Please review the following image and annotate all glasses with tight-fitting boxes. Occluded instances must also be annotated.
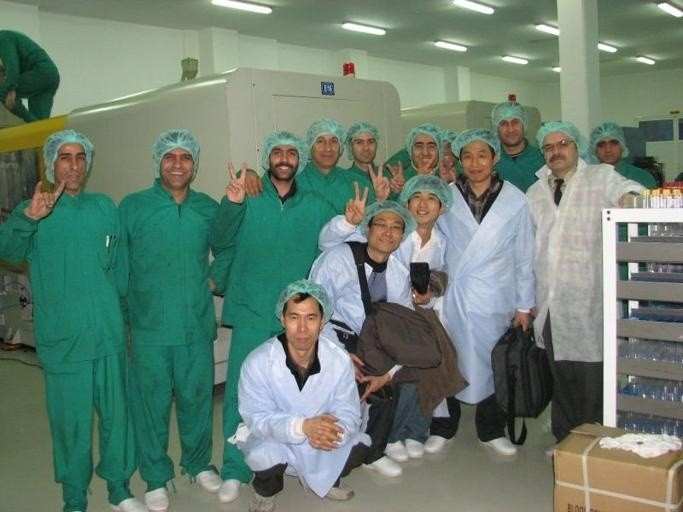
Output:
[542,139,575,153]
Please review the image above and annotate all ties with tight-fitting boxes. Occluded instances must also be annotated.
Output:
[554,179,564,206]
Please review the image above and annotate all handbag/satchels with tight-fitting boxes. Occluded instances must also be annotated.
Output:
[354,314,420,386]
[490,316,553,419]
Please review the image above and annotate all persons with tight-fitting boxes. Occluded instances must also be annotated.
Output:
[0,128,150,511]
[214,133,342,501]
[225,280,363,512]
[116,128,236,512]
[0,29,60,124]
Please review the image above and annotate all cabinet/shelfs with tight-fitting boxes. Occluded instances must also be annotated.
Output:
[600,205,682,440]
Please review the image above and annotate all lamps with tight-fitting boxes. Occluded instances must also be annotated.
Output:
[635,56,656,66]
[210,1,273,15]
[535,23,560,36]
[597,42,618,54]
[656,1,682,18]
[552,65,561,72]
[433,40,467,52]
[341,21,387,36]
[453,0,495,15]
[502,55,529,65]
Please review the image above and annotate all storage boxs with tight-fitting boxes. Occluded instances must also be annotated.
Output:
[550,422,682,512]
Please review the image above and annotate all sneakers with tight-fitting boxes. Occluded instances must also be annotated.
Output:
[217,471,255,503]
[424,435,455,456]
[405,438,425,458]
[325,478,356,502]
[195,462,223,493]
[248,490,280,512]
[104,492,150,512]
[384,440,408,462]
[361,453,403,478]
[143,487,169,512]
[476,434,518,457]
